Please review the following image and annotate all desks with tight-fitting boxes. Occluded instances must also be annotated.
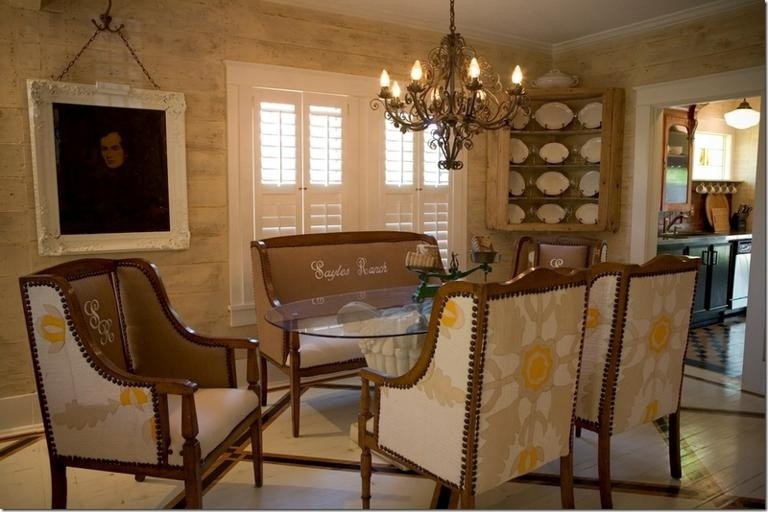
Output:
[264,282,476,472]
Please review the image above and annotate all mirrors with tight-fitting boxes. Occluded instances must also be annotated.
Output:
[660,109,691,213]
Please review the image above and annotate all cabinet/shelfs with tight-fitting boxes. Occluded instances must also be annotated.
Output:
[688,243,733,327]
[485,85,627,234]
[658,248,688,255]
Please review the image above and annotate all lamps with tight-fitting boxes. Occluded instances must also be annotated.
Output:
[724,98,760,129]
[368,0,531,172]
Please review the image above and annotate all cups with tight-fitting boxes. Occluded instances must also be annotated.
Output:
[695,182,737,194]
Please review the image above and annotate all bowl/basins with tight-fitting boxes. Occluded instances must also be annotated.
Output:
[669,146,684,155]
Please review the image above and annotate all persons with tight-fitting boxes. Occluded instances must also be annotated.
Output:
[81,129,161,232]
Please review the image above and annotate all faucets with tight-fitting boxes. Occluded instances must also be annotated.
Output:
[661,211,688,233]
[662,236,688,241]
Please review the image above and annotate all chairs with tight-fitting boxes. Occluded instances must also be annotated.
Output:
[507,236,606,278]
[356,266,587,510]
[20,260,263,510]
[576,253,701,509]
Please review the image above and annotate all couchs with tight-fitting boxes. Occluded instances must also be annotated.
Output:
[250,230,447,438]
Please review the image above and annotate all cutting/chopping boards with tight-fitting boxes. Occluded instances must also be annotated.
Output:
[711,207,732,233]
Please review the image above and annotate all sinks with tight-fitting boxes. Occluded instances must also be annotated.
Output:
[658,231,712,239]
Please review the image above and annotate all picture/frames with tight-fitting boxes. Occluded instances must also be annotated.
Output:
[26,80,191,256]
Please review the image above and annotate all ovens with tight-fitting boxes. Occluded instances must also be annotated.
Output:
[727,238,752,310]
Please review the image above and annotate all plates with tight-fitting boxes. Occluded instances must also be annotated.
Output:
[506,101,603,223]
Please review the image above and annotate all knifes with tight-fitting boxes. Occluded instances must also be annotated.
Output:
[735,202,753,218]
